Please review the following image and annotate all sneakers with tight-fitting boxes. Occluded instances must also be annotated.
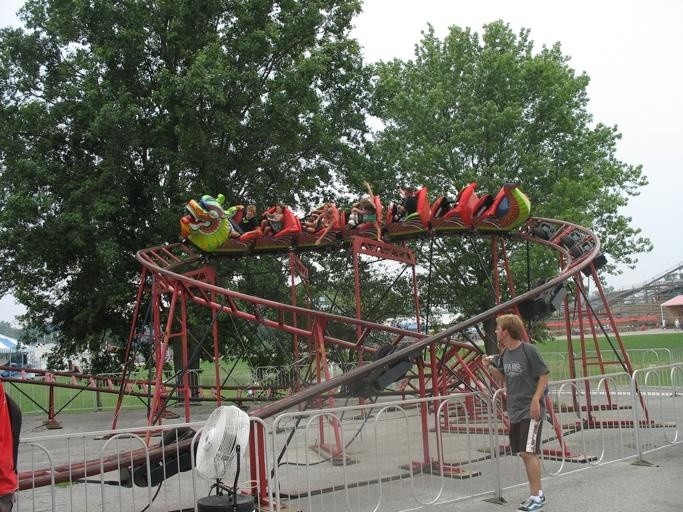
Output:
[516,496,546,512]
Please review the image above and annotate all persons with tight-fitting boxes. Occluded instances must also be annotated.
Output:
[349,184,418,228]
[262,203,284,236]
[234,204,260,239]
[302,203,334,233]
[480,314,550,511]
